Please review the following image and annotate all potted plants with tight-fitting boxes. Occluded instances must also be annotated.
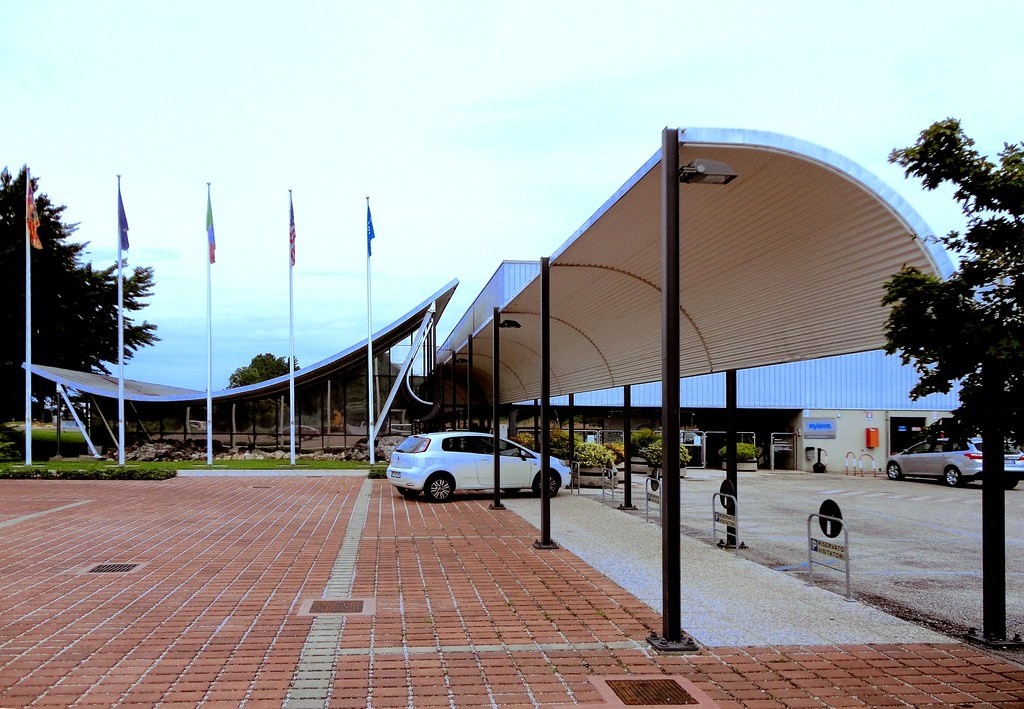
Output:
[570,428,692,487]
[717,441,763,471]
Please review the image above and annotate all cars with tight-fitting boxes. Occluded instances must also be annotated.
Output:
[386,431,572,504]
[271,425,320,441]
[886,436,1024,490]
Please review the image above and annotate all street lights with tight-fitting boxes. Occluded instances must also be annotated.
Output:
[646,127,735,653]
[450,352,466,432]
[486,307,521,509]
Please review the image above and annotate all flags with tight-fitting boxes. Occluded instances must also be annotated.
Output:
[206,195,216,264]
[367,203,375,258]
[119,191,129,251]
[289,191,296,267]
[25,173,42,249]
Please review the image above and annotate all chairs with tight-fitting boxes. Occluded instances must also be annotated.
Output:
[942,444,954,451]
